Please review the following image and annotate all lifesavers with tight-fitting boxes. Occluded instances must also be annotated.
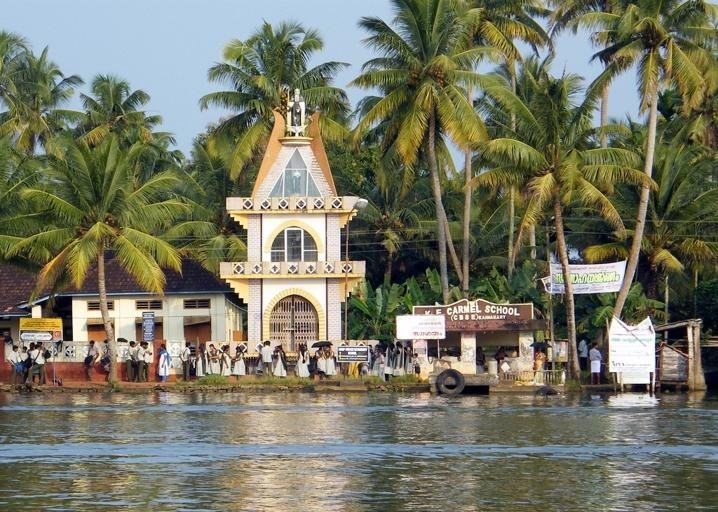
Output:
[436,369,464,395]
